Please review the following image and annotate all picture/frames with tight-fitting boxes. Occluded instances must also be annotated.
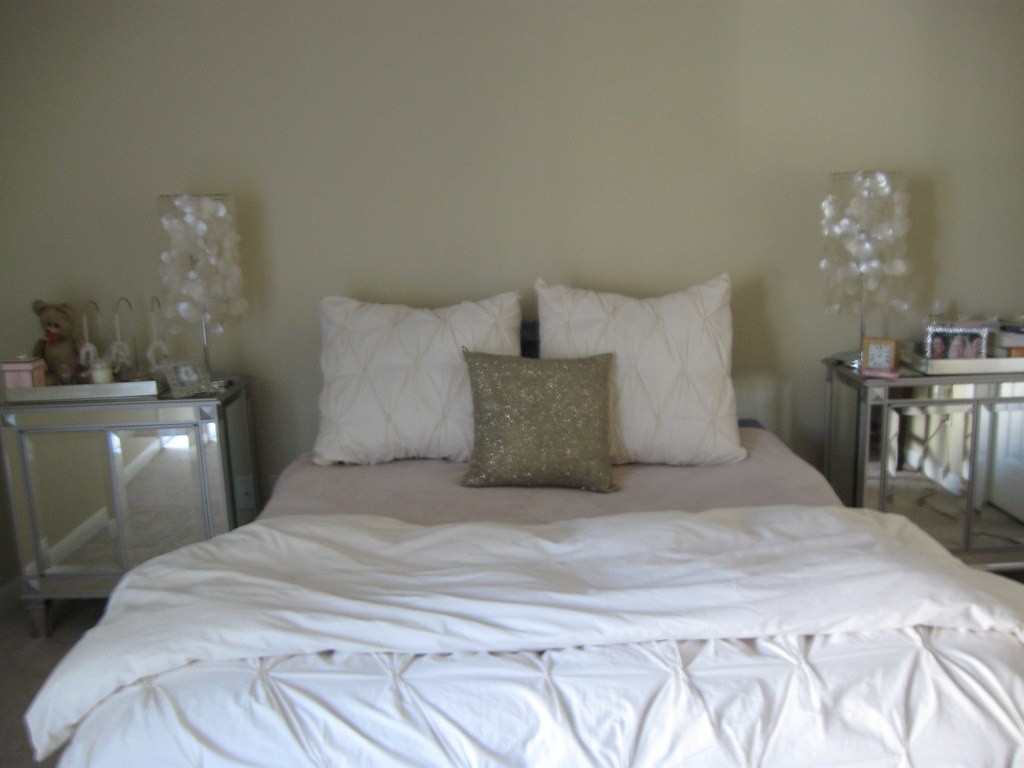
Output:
[924,326,988,360]
[160,353,213,398]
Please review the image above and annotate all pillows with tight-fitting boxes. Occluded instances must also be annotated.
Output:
[532,272,751,468]
[459,344,622,494]
[309,289,523,468]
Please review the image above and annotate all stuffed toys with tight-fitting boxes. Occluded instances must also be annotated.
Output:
[32,300,82,386]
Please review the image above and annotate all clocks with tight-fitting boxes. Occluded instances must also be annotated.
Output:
[860,337,898,372]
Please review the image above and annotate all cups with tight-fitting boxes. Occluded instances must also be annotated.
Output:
[88,358,114,384]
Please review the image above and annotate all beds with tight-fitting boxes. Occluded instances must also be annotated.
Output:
[21,374,1024,768]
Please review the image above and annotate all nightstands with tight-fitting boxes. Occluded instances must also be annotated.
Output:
[0,376,262,639]
[818,348,1023,573]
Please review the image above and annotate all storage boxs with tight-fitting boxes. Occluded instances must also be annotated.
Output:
[0,354,47,387]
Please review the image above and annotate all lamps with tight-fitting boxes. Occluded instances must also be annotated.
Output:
[157,190,250,388]
[815,171,912,367]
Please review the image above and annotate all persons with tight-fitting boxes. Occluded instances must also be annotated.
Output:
[931,333,982,358]
